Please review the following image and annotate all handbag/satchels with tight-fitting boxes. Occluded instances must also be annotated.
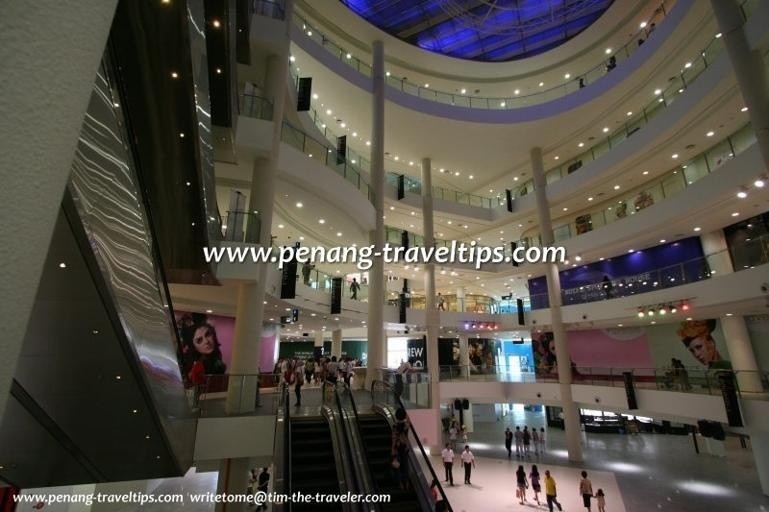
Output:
[516,487,521,499]
[296,368,304,385]
[535,484,541,492]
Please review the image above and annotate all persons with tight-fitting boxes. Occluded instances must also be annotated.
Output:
[189,323,226,384]
[246,468,257,495]
[438,293,445,311]
[391,408,412,490]
[442,421,605,512]
[256,355,362,408]
[256,467,269,512]
[302,258,315,284]
[533,333,557,373]
[395,357,417,405]
[350,277,361,300]
[676,319,723,369]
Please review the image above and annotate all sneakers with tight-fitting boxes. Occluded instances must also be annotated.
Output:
[294,401,301,406]
